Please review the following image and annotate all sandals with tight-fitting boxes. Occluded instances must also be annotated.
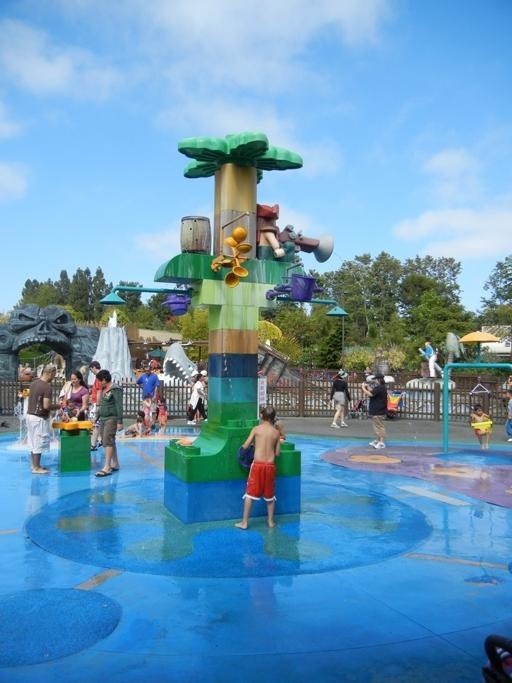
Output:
[91,445,97,451]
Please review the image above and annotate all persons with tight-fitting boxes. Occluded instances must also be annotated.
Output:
[329,370,352,430]
[16,358,209,453]
[504,389,512,443]
[480,630,512,683]
[419,341,433,361]
[361,373,388,450]
[233,402,278,529]
[24,360,63,475]
[469,404,492,452]
[92,370,125,477]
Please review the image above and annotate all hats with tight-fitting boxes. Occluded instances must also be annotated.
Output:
[371,374,384,380]
[338,371,348,378]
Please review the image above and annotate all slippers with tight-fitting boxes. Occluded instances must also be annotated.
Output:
[95,470,112,476]
[110,466,119,471]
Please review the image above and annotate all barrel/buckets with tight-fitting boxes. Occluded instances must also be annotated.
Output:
[179,215,212,255]
[291,273,317,302]
[238,444,255,469]
[167,293,188,316]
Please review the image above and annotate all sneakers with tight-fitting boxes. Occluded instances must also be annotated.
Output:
[374,442,385,448]
[341,422,348,427]
[187,420,196,424]
[330,423,340,429]
[369,440,378,446]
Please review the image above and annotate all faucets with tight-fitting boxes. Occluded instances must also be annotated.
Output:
[221,211,250,229]
